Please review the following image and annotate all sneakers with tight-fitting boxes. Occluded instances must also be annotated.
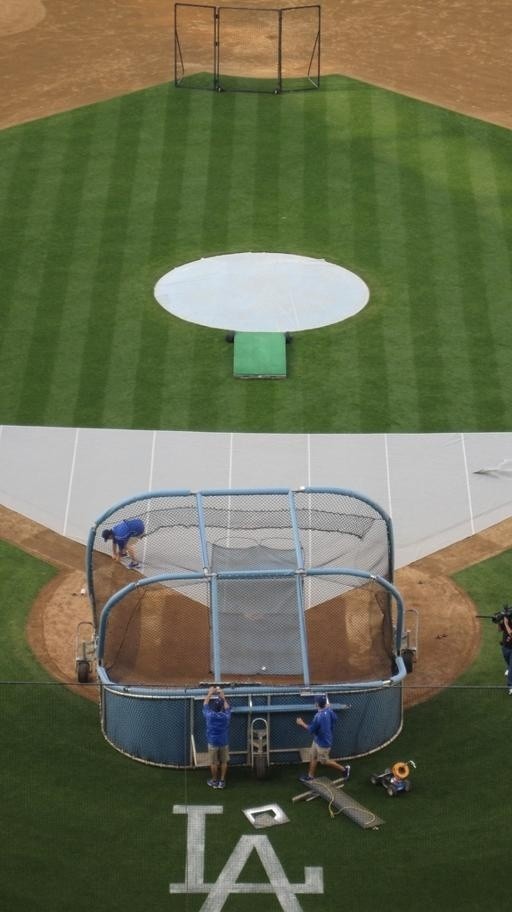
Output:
[122,552,129,558]
[298,775,315,782]
[218,780,225,789]
[127,561,142,569]
[342,765,351,779]
[206,778,218,788]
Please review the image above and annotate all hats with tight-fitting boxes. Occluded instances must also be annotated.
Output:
[213,697,225,708]
[314,693,327,705]
[101,528,114,541]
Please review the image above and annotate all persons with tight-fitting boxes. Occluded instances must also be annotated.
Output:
[296,693,350,783]
[202,686,231,790]
[101,517,145,570]
[497,606,512,697]
